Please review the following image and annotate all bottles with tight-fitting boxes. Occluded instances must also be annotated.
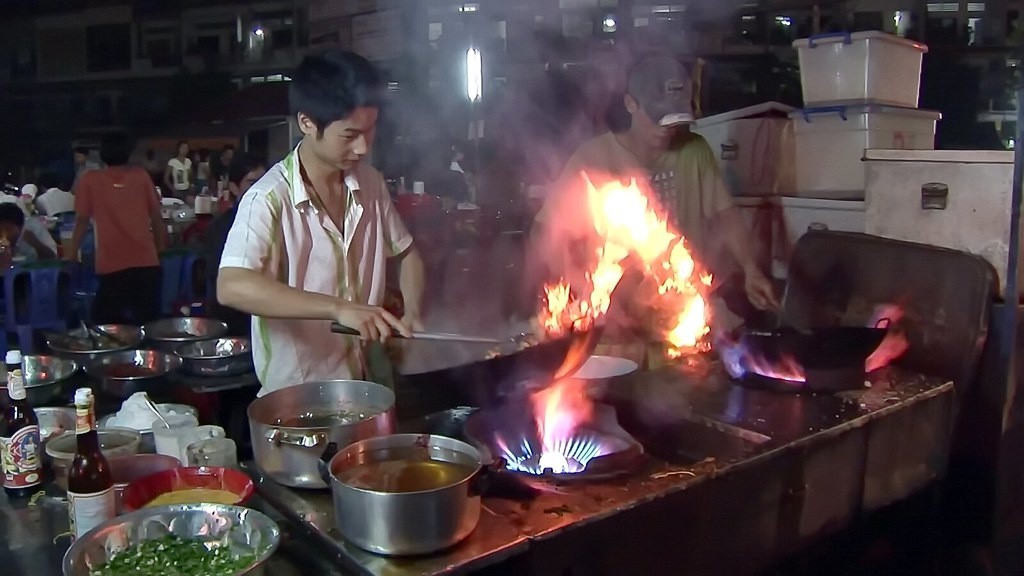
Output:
[67,388,116,544]
[0,228,12,274]
[0,351,46,499]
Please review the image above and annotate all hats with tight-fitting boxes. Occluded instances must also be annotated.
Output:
[628,53,696,128]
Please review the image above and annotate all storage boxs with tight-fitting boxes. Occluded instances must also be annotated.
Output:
[691,31,1018,305]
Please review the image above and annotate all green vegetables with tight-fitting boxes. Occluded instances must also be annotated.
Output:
[88,532,255,576]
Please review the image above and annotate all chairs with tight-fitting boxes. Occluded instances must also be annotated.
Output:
[0,207,201,352]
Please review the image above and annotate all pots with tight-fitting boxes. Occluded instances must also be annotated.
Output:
[247,380,397,489]
[719,318,891,384]
[318,432,502,555]
[398,318,606,408]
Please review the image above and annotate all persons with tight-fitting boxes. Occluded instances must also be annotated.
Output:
[216,45,432,397]
[0,104,523,371]
[527,55,780,330]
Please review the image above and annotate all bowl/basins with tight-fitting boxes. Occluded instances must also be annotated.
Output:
[1,356,78,405]
[47,424,142,491]
[120,466,254,512]
[62,502,279,576]
[96,405,198,454]
[173,338,254,376]
[82,348,182,401]
[145,317,229,354]
[45,324,144,365]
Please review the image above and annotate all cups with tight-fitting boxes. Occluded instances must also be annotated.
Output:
[178,425,225,466]
[186,439,237,466]
[222,189,231,202]
[153,415,199,462]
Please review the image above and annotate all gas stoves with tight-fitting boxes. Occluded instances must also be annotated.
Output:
[395,295,953,539]
